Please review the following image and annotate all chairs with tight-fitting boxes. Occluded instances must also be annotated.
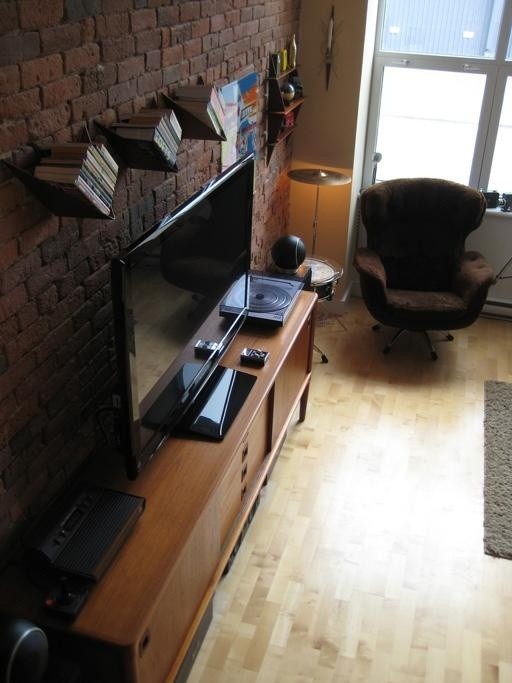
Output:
[352,177,494,362]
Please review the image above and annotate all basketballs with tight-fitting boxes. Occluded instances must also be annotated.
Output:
[272,235,305,269]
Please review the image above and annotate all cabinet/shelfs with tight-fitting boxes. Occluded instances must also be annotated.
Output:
[267,50,306,166]
[32,276,320,683]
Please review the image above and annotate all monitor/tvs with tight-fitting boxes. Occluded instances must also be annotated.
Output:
[109,151,258,482]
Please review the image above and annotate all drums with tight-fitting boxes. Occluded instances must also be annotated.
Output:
[271,254,343,303]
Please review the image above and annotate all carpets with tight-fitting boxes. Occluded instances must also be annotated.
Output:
[483,380,512,561]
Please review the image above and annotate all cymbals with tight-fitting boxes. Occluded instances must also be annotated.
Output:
[287,169,352,186]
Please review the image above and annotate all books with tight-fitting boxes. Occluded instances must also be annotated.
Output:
[29,82,227,216]
